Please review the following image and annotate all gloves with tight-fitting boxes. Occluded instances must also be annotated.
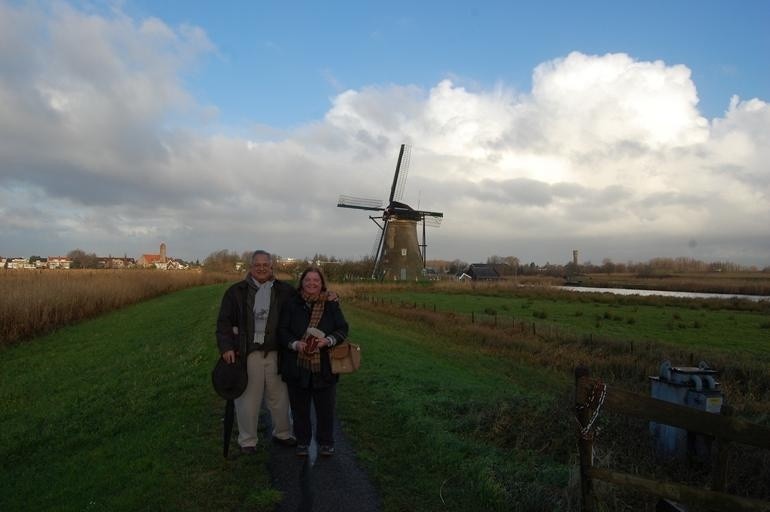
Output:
[303,327,326,355]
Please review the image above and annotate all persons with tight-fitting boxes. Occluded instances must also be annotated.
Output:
[275,266,350,457]
[215,250,341,456]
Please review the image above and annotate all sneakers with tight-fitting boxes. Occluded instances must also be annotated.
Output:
[238,434,337,457]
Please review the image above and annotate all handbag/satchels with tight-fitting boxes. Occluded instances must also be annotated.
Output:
[211,352,248,401]
[328,337,361,374]
